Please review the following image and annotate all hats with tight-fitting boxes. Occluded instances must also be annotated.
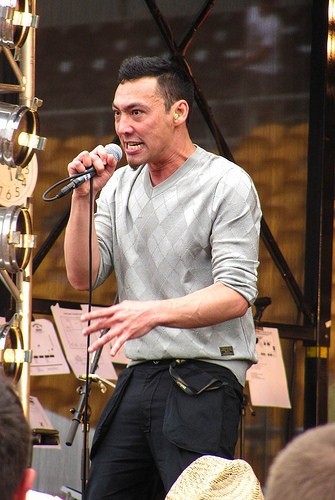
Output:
[165,455,264,500]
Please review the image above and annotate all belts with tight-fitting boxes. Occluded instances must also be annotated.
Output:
[148,360,224,369]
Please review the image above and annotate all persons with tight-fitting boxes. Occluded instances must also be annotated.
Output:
[64,52,263,500]
[228,0,288,121]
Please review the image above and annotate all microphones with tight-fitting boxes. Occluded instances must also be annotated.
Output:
[56,143,123,199]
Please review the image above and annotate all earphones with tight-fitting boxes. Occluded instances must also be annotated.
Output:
[174,112,179,120]
